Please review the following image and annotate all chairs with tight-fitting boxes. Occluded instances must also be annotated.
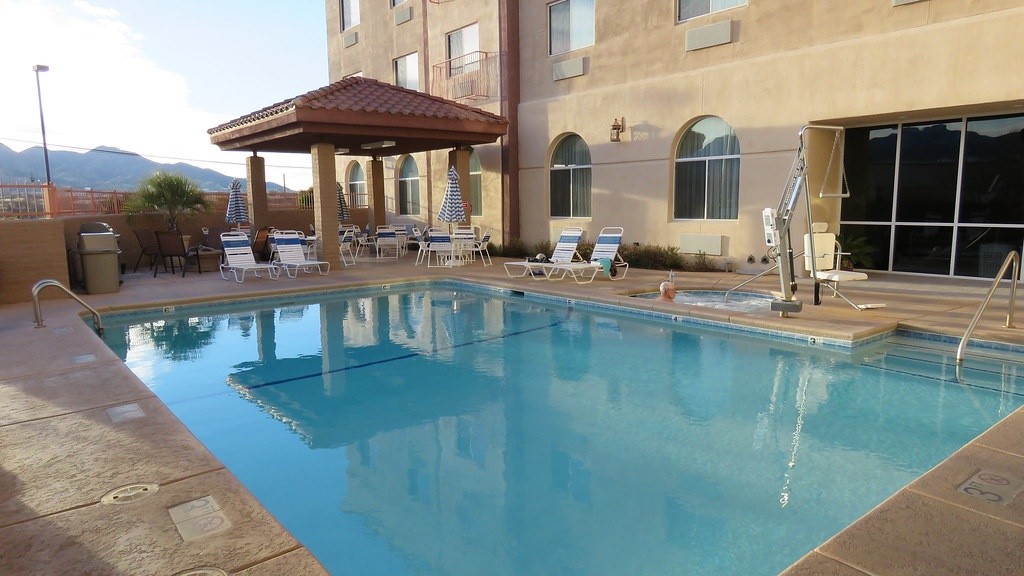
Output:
[804,222,887,312]
[154,231,202,277]
[504,227,588,281]
[542,226,629,285]
[220,224,493,283]
[132,229,183,273]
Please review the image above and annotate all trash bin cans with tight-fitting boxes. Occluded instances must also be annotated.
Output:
[75,231,122,295]
[77,221,124,285]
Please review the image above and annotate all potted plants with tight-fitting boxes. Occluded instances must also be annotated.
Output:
[123,172,215,268]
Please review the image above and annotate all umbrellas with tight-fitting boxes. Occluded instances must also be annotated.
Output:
[225,177,249,232]
[336,182,350,227]
[437,166,466,235]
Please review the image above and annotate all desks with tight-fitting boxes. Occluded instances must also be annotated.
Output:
[183,251,222,272]
[432,235,476,266]
[301,236,316,259]
[375,231,407,257]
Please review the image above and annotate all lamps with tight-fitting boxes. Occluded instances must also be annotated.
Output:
[610,116,624,142]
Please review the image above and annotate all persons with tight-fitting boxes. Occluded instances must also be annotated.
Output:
[656,282,676,303]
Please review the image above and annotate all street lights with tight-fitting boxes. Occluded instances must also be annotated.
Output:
[32,64,51,185]
